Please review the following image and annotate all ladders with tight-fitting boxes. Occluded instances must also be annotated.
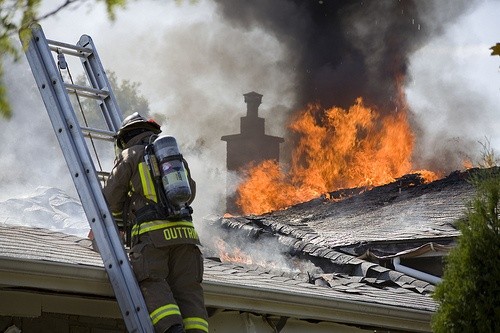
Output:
[18,22,154,333]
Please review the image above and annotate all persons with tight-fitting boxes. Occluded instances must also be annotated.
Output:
[102,111,212,332]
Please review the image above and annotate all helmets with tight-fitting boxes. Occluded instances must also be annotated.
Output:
[112,111,162,150]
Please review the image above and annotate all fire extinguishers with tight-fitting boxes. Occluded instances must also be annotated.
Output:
[145,134,193,217]
